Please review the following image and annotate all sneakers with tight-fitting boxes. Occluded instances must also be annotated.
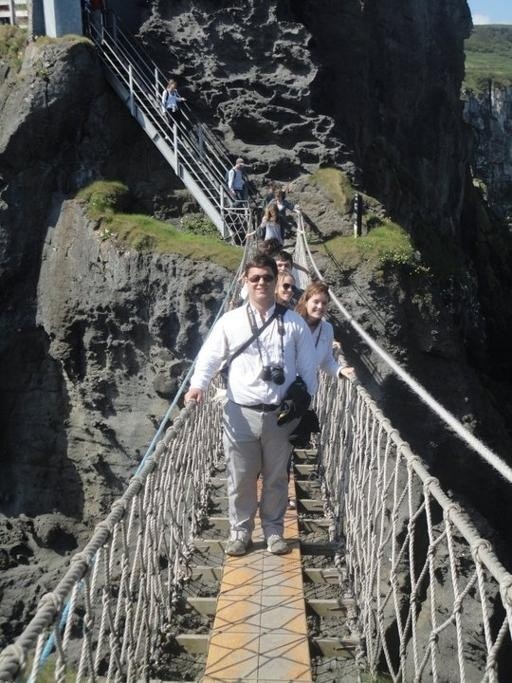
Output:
[265,532,289,554]
[224,530,252,556]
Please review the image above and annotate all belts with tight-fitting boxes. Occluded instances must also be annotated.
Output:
[231,404,279,413]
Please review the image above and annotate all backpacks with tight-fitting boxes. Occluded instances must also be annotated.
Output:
[224,169,236,185]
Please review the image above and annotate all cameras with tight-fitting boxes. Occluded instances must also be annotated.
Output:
[262,365,285,386]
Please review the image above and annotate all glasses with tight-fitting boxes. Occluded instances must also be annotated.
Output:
[280,283,297,292]
[247,275,276,283]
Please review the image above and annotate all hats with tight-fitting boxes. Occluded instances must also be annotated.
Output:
[237,158,245,166]
[276,385,311,427]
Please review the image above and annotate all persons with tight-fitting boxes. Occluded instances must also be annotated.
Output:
[245,206,305,254]
[161,79,187,140]
[259,185,283,216]
[272,252,293,276]
[273,273,297,313]
[266,191,298,241]
[183,255,321,555]
[89,0,106,46]
[228,158,246,212]
[266,284,355,510]
[258,239,312,277]
